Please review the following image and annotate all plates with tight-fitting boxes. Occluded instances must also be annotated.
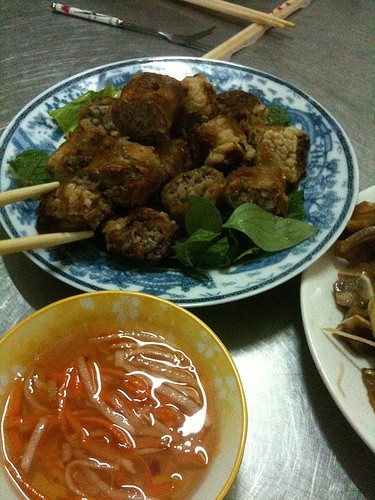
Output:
[299,183,375,455]
[1,56,361,308]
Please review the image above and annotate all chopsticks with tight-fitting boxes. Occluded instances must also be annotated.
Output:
[199,0,308,61]
[183,0,295,29]
[1,181,95,258]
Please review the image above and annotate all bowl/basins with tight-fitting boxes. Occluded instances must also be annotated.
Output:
[0,290,247,500]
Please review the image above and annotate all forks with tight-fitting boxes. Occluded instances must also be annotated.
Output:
[51,3,218,47]
